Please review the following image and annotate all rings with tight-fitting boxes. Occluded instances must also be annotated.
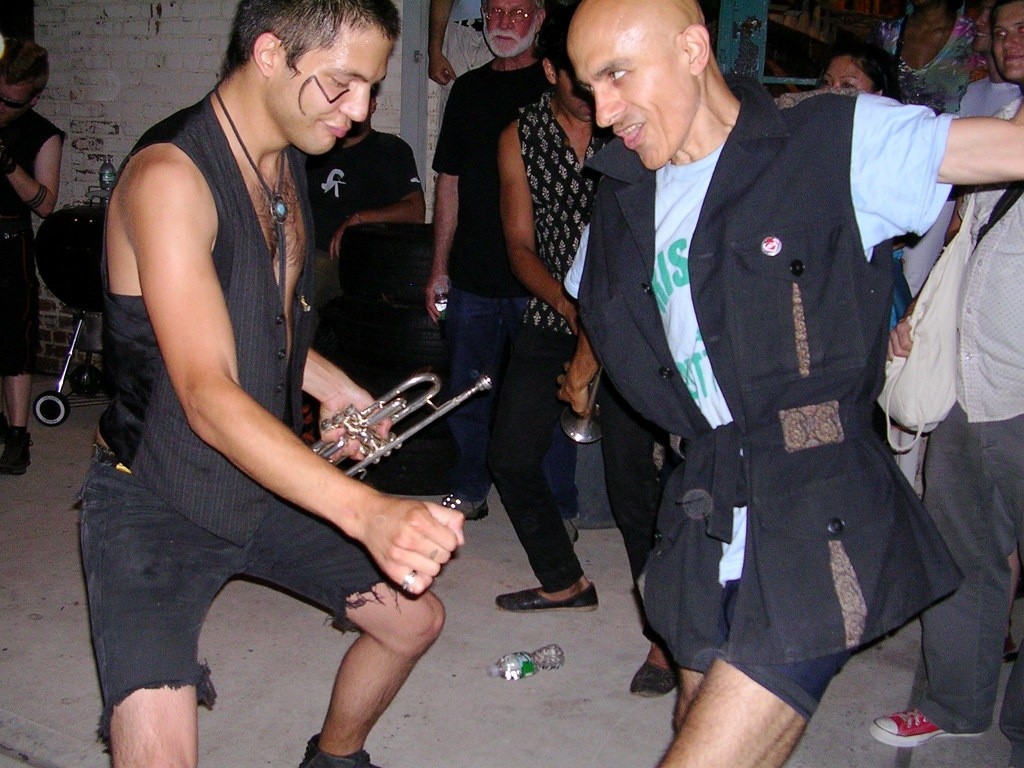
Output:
[430,547,439,560]
[401,570,417,591]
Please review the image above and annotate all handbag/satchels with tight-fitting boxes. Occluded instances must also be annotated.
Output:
[877,190,976,453]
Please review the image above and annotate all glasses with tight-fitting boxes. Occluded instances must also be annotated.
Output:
[0,88,39,108]
[484,7,538,22]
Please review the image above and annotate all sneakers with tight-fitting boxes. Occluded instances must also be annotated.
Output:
[0,411,9,444]
[869,707,984,747]
[0,429,33,475]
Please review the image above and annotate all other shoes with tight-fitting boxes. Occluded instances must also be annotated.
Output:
[495,581,599,613]
[562,517,579,548]
[446,499,489,521]
[630,660,677,697]
[298,732,381,768]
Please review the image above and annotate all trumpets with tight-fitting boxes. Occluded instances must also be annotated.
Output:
[308,369,494,478]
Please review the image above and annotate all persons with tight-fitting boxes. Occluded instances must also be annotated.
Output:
[0,38,68,474]
[76,1,464,768]
[569,1,1024,768]
[302,1,685,696]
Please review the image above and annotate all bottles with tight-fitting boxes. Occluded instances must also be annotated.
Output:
[433,284,448,342]
[486,642,565,681]
[99,155,117,206]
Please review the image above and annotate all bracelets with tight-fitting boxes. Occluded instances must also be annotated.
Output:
[354,213,362,226]
[0,156,17,174]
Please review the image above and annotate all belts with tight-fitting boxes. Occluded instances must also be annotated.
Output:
[457,19,482,32]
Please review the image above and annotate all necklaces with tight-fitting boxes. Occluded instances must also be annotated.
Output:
[215,83,287,224]
